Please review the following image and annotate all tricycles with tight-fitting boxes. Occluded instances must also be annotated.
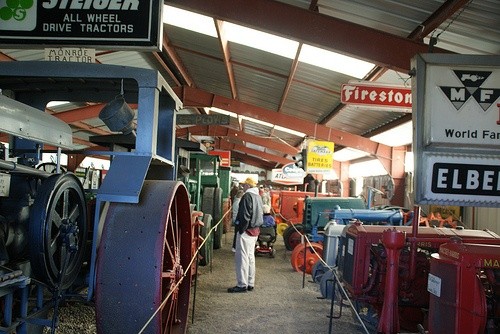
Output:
[256,222,276,258]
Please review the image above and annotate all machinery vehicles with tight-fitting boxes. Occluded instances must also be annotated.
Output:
[259,179,500,334]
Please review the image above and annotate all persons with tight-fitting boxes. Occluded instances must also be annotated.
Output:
[228,177,263,292]
[232,190,243,252]
[230,180,272,226]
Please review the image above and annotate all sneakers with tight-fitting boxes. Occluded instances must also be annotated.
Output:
[231,248,236,253]
[227,286,254,293]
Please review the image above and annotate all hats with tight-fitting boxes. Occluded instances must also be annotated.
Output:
[263,205,270,213]
[239,178,256,186]
